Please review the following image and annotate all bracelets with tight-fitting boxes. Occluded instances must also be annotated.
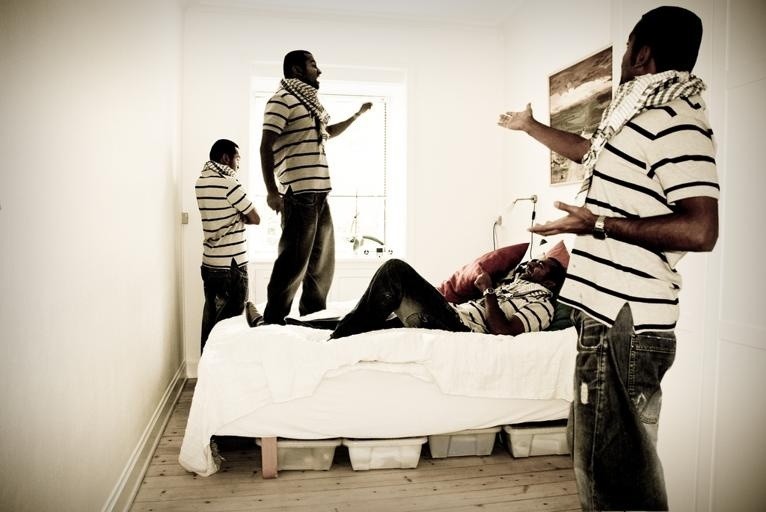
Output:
[355,113,361,116]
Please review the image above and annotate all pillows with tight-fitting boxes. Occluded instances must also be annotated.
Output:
[438,240,572,304]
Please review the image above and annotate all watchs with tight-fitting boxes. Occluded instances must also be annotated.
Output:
[592,215,607,240]
[483,287,496,296]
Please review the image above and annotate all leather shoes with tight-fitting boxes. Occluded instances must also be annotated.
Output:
[246,302,263,327]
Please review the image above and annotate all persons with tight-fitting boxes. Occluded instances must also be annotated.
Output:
[499,4,723,512]
[244,257,567,341]
[193,136,261,359]
[262,48,376,327]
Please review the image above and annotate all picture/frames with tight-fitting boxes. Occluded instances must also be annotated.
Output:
[546,45,613,187]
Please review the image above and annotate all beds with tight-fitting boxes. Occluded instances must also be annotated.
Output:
[193,303,577,479]
